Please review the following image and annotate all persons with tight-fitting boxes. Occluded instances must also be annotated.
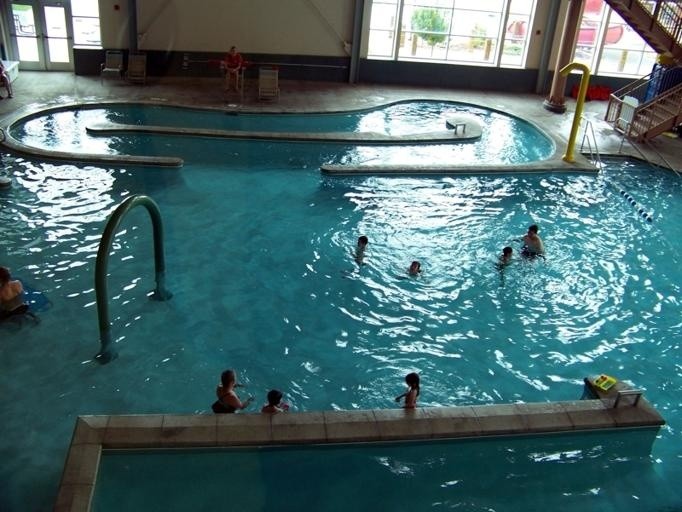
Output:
[395,261,423,280]
[261,389,291,413]
[1,266,42,330]
[494,246,521,287]
[211,368,256,413]
[223,46,242,94]
[339,235,368,280]
[393,371,421,408]
[0,58,14,99]
[509,223,549,266]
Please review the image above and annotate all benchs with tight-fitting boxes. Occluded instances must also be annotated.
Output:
[572,82,612,100]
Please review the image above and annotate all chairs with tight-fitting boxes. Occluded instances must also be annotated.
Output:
[258,68,280,103]
[125,56,146,85]
[220,60,248,96]
[100,51,124,86]
